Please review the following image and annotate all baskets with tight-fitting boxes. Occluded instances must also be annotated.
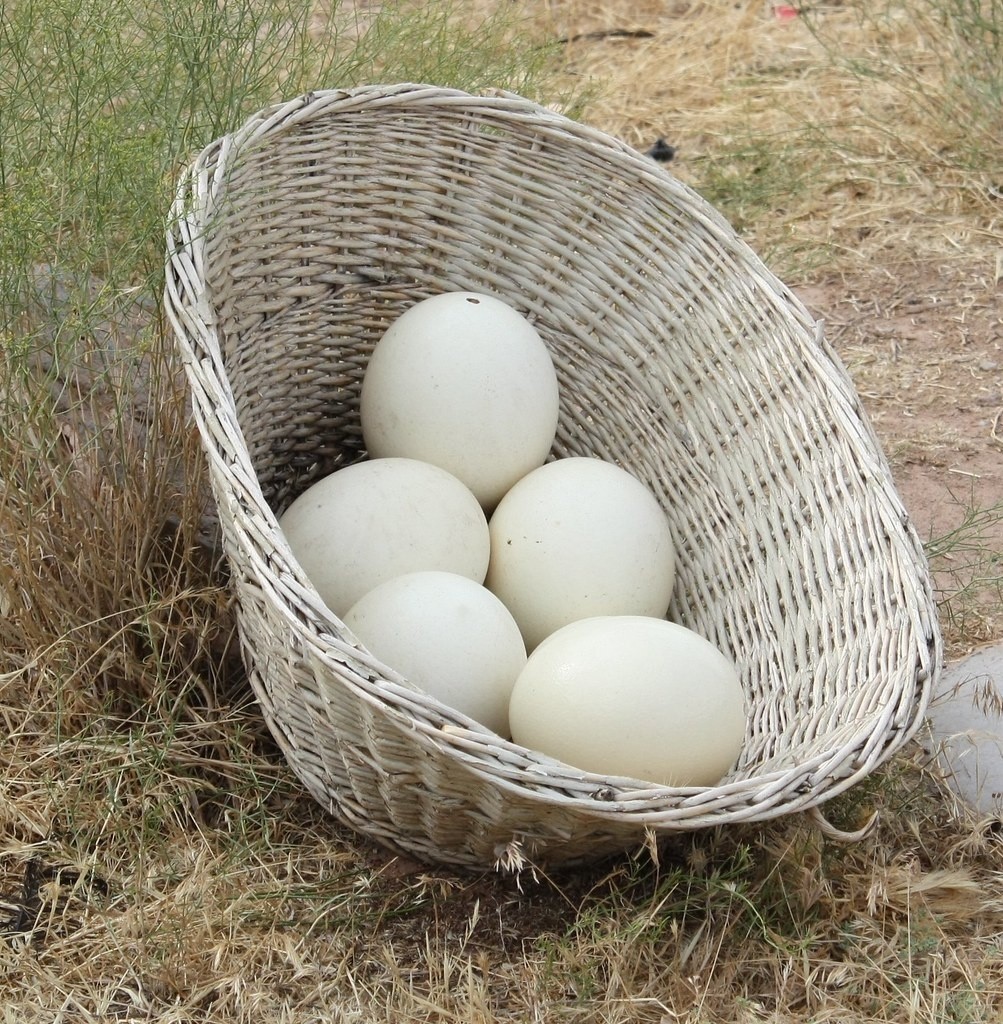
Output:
[163,83,943,875]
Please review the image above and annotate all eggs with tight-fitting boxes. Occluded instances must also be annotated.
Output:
[279,291,746,786]
